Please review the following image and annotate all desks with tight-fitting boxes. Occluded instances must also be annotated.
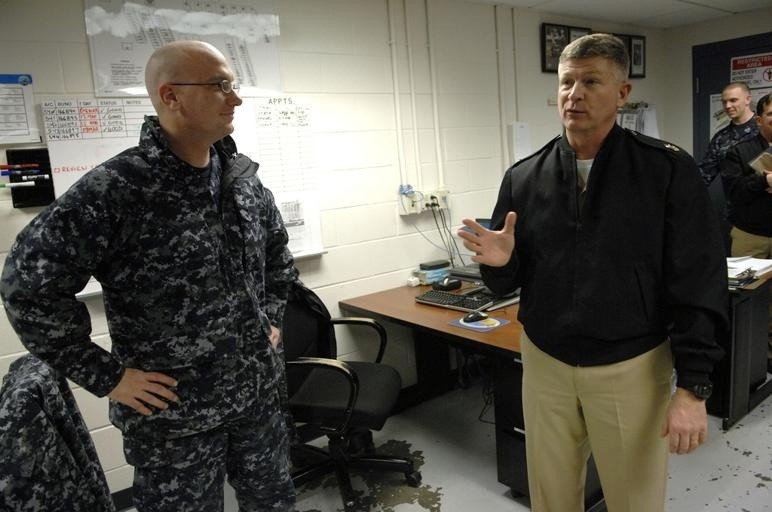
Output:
[338,280,607,512]
[706,255,772,431]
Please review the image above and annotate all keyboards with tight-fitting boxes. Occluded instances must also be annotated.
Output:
[414,289,494,313]
[469,292,520,311]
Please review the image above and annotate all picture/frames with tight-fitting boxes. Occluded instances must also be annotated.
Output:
[540,22,646,79]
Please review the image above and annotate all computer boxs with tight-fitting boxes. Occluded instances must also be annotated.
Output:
[493,375,602,504]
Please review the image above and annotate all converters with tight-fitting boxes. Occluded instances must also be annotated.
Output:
[407,276,421,287]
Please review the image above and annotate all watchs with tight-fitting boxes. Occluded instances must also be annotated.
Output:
[677,381,711,400]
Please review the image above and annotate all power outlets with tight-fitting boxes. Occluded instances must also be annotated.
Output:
[422,192,440,212]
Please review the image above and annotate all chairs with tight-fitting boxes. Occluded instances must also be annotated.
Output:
[284,283,422,512]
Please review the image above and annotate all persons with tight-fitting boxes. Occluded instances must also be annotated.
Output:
[457,31,728,511]
[698,83,761,206]
[723,95,772,259]
[0,38,299,511]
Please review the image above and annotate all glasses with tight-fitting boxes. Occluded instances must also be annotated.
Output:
[166,76,235,95]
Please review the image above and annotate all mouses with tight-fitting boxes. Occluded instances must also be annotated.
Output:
[463,310,488,322]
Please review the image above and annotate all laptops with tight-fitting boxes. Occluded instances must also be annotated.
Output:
[450,218,492,279]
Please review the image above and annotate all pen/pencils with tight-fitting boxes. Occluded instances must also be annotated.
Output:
[0,164,50,188]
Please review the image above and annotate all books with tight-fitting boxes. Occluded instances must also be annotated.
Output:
[725,256,772,291]
